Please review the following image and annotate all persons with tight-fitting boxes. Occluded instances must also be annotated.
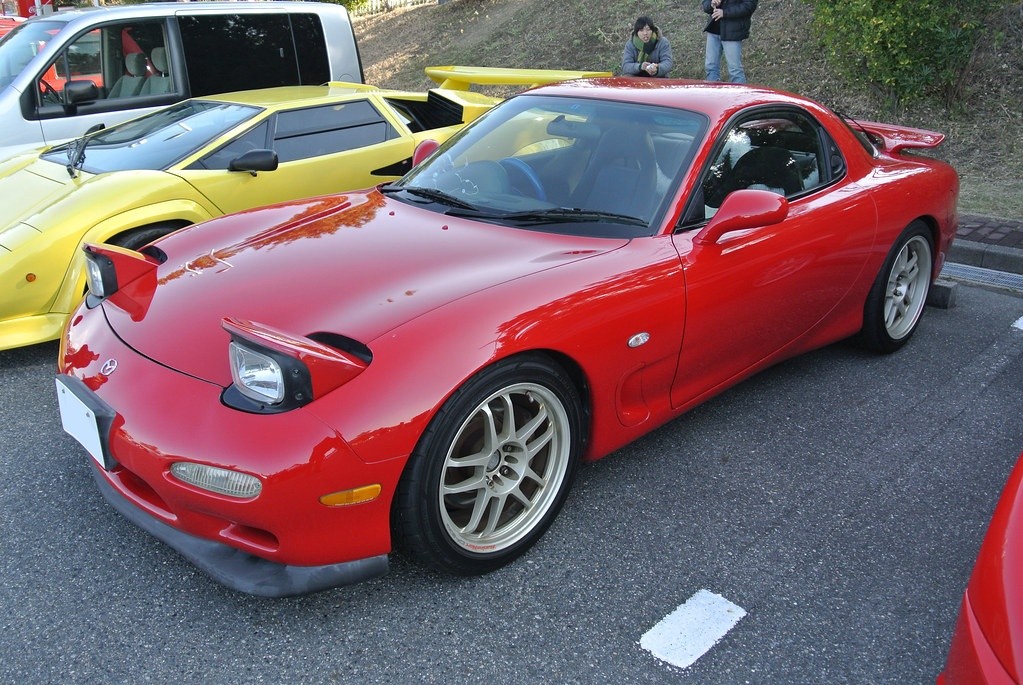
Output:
[622,16,672,79]
[702,0,760,85]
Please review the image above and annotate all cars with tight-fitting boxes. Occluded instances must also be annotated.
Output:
[54,76,958,598]
[0,66,612,351]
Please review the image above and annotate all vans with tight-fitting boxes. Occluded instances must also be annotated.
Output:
[0,2,365,147]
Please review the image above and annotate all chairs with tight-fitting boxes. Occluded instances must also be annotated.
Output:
[139,47,171,96]
[565,125,659,223]
[107,53,146,100]
[706,147,805,208]
